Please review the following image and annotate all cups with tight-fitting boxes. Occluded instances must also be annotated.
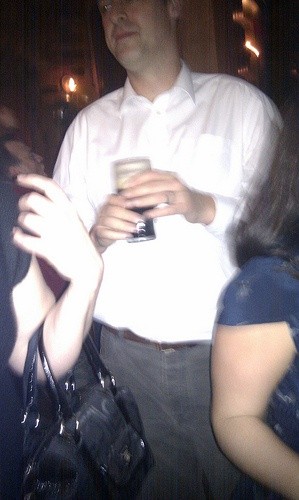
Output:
[111,157,156,243]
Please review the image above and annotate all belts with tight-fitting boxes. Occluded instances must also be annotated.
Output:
[101,325,198,350]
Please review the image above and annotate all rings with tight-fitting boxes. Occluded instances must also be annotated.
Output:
[164,191,176,205]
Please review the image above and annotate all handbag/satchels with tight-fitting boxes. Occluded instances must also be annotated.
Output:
[15,319,153,499]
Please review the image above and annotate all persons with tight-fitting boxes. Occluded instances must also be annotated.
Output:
[1,114,104,500]
[211,93,299,499]
[54,1,282,499]
